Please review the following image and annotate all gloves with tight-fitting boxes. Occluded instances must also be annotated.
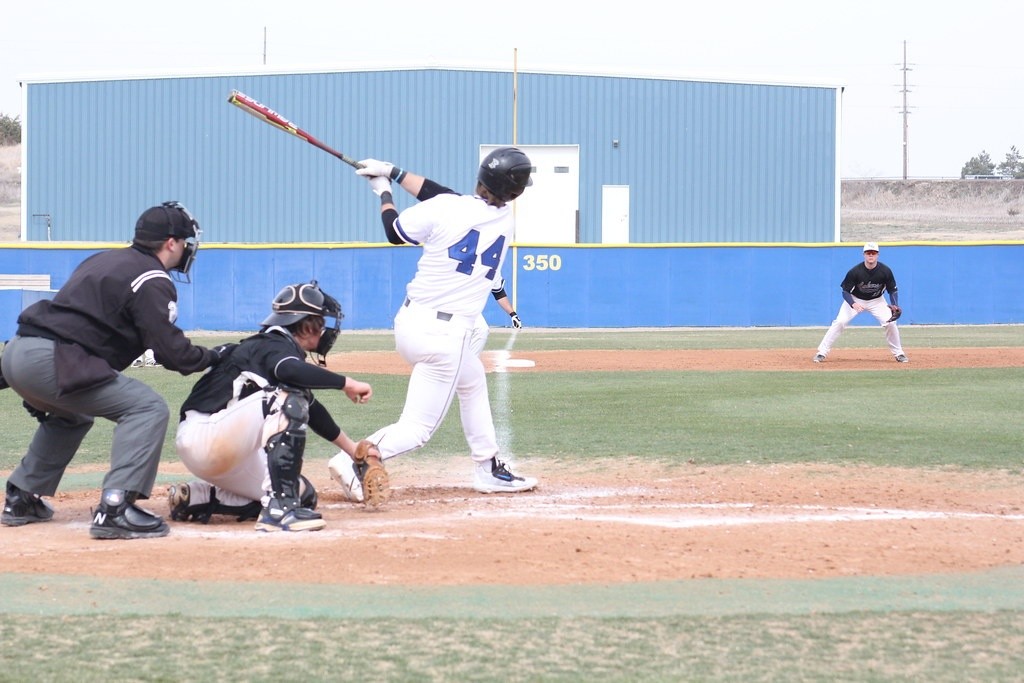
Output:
[355,158,408,186]
[510,312,522,329]
[207,343,240,366]
[367,175,393,206]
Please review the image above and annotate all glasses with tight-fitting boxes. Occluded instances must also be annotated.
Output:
[864,251,878,256]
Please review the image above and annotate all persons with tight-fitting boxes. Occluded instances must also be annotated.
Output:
[329,147,538,502]
[813,242,909,363]
[132,349,162,367]
[166,280,372,532]
[0,202,239,539]
[468,275,521,357]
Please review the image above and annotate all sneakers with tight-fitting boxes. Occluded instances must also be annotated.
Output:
[1,487,54,526]
[254,505,326,533]
[813,353,825,363]
[170,484,191,519]
[90,492,170,540]
[473,461,538,495]
[329,449,363,502]
[896,355,908,363]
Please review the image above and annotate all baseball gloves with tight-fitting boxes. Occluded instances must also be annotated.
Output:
[351,439,392,506]
[886,304,902,323]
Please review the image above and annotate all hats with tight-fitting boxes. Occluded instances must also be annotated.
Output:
[136,205,195,240]
[863,242,879,253]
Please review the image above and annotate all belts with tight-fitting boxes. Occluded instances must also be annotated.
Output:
[406,299,453,322]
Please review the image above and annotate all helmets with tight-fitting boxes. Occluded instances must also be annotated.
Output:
[259,283,328,327]
[479,147,533,202]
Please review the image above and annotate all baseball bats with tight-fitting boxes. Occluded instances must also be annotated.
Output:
[225,88,393,186]
